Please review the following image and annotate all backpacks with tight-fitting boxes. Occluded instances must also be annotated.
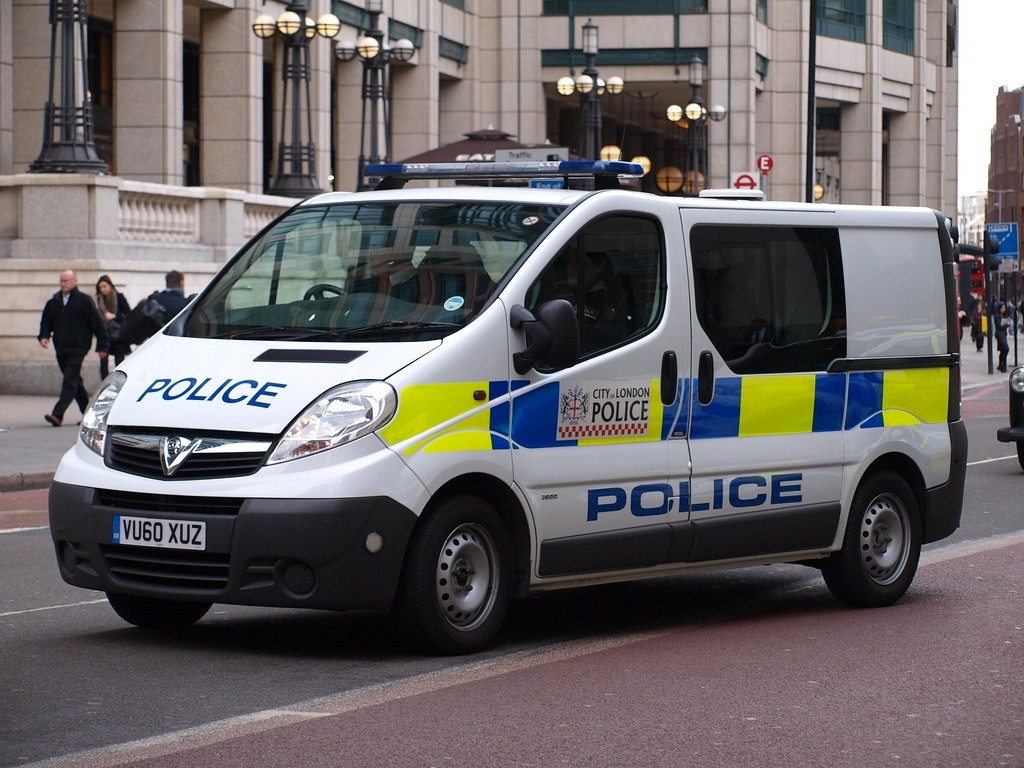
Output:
[123,298,167,346]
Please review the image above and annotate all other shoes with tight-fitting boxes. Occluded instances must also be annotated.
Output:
[44,414,61,426]
[976,349,983,353]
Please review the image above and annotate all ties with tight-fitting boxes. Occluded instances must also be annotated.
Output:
[754,332,761,343]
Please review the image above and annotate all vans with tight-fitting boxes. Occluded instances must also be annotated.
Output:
[48,161,969,657]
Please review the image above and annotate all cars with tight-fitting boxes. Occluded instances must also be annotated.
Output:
[996,364,1024,476]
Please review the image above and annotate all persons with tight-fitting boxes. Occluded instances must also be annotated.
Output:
[147,271,187,338]
[96,275,129,381]
[38,270,107,428]
[957,295,1024,352]
[994,304,1010,373]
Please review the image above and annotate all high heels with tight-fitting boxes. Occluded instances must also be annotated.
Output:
[997,366,1002,373]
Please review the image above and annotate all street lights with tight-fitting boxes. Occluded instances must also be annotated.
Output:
[249,1,343,199]
[559,21,627,162]
[665,53,729,193]
[335,0,421,190]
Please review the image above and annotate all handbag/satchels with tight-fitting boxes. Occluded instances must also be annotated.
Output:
[108,312,125,339]
